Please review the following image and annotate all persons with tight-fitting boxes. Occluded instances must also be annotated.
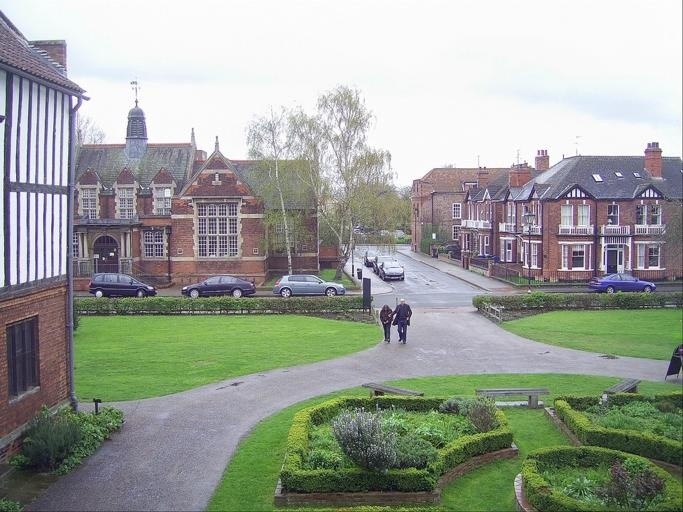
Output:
[379,304,394,342]
[388,299,412,345]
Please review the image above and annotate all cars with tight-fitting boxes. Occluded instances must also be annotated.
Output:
[272,274,346,298]
[590,272,657,294]
[364,250,405,281]
[181,274,257,299]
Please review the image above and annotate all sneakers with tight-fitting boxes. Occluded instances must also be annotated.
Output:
[384,339,390,342]
[399,338,406,344]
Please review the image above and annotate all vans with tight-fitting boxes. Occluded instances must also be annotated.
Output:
[87,271,158,298]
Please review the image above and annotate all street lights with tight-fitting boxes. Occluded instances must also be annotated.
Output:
[523,208,536,294]
